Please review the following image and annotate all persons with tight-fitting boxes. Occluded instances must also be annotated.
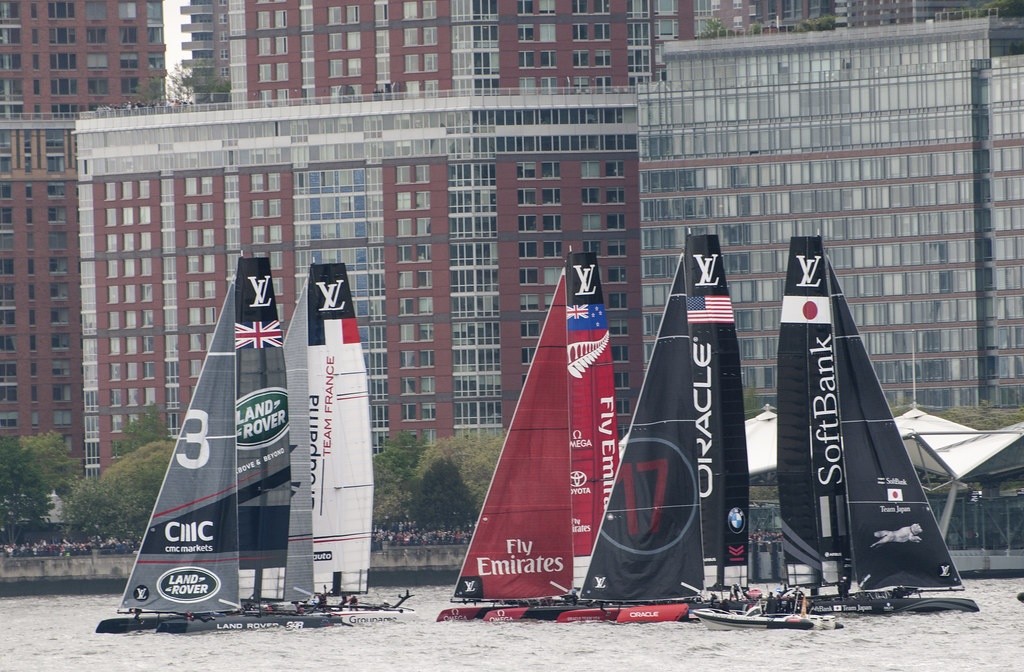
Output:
[338,596,347,610]
[793,586,804,597]
[185,612,215,623]
[347,596,358,611]
[757,593,762,598]
[711,592,717,602]
[570,591,579,606]
[754,528,783,552]
[319,595,326,605]
[768,592,782,606]
[0,537,141,557]
[296,604,305,614]
[730,583,743,601]
[837,577,849,600]
[372,520,472,546]
[134,609,142,619]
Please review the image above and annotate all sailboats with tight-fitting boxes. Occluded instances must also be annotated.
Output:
[434,229,980,622]
[95,256,345,634]
[280,262,417,626]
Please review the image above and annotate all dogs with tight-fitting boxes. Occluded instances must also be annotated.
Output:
[873,523,923,545]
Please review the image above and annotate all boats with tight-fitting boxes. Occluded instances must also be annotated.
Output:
[691,583,844,631]
